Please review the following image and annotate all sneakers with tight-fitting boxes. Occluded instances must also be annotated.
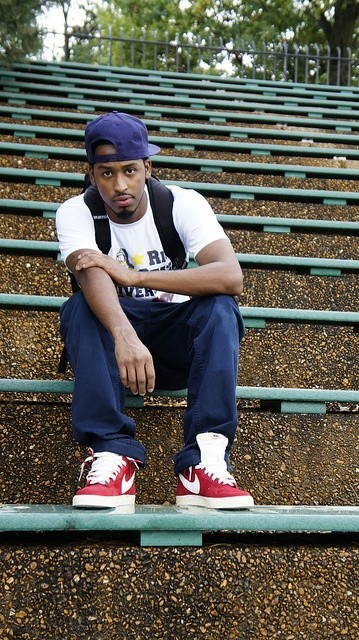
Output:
[175,431,254,510]
[71,452,136,507]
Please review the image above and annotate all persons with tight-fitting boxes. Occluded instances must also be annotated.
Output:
[55,111,256,510]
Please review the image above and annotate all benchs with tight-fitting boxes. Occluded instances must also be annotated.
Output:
[0,56,359,548]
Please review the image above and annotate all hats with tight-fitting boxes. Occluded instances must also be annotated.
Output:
[84,113,162,164]
